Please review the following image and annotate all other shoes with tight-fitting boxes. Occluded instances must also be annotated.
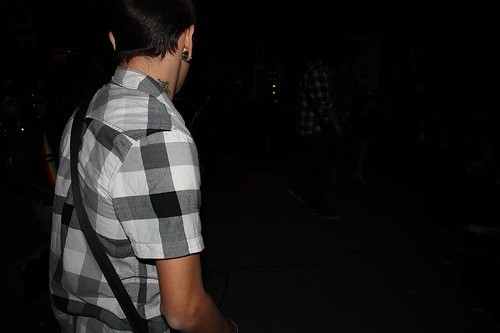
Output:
[288,183,311,205]
[308,199,341,219]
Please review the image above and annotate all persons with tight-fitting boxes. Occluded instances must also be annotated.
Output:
[18,43,64,293]
[289,43,356,220]
[46,1,238,333]
